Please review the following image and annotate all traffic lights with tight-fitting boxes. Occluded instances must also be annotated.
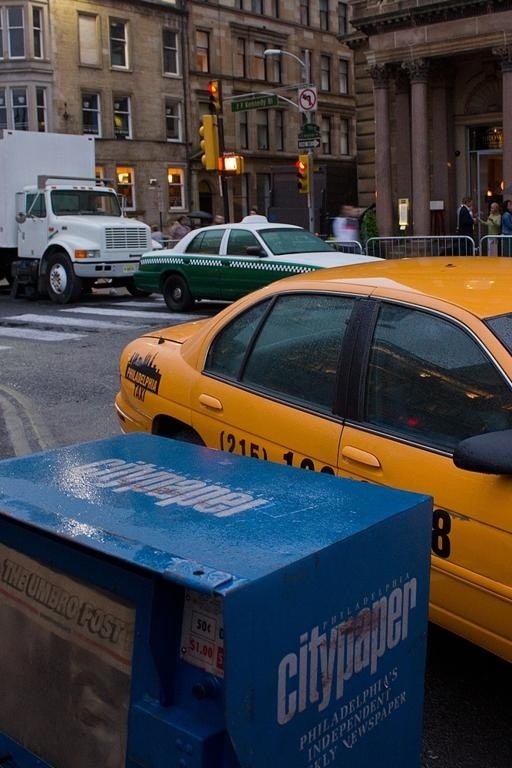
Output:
[207,80,224,113]
[198,116,219,170]
[222,156,241,177]
[295,155,310,193]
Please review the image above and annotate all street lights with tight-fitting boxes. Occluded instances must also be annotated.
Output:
[262,48,316,231]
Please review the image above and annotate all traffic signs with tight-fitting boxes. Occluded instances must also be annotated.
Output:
[298,123,321,149]
[230,94,281,113]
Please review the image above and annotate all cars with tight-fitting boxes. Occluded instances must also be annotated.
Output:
[114,255,512,664]
[132,216,387,311]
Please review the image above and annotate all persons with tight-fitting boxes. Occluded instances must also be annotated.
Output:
[456,196,512,258]
[333,203,362,256]
[211,213,226,226]
[168,216,191,250]
[249,208,258,216]
[149,224,172,250]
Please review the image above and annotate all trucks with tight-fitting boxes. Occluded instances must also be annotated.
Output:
[1,129,165,305]
